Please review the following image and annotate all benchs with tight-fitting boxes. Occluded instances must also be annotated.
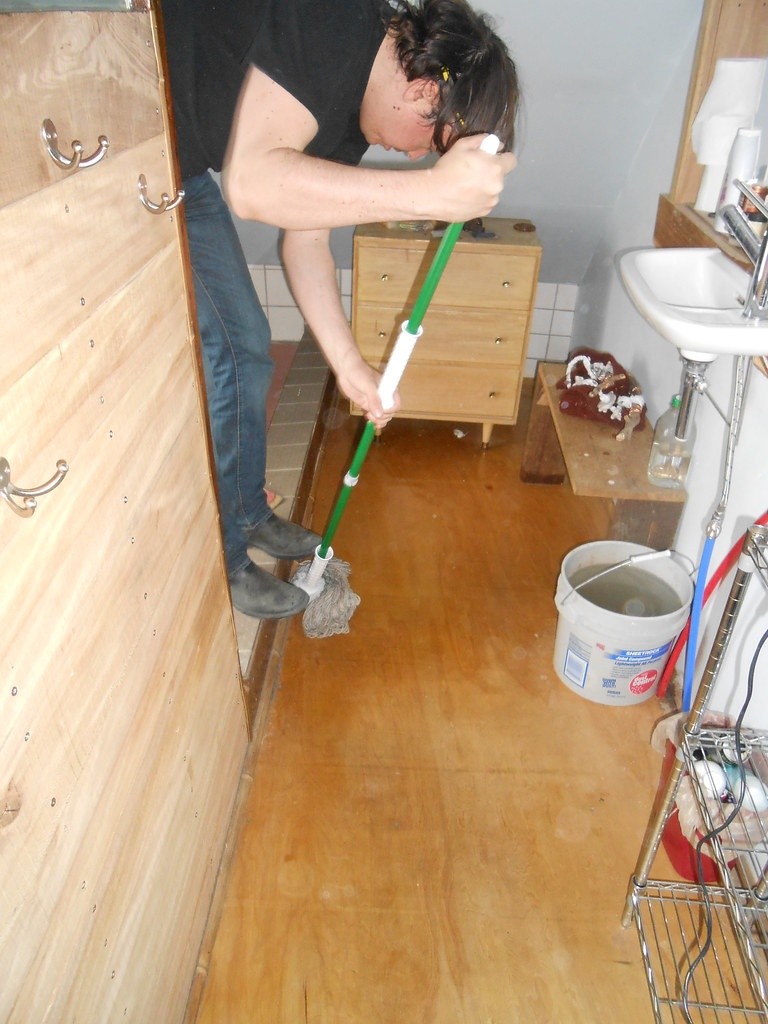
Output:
[520,362,689,551]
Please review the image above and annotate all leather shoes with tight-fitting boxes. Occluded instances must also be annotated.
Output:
[228,561,310,619]
[246,513,323,559]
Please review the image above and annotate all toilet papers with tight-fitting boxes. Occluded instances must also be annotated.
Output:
[690,56,765,214]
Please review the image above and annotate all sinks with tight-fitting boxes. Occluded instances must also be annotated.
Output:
[612,245,767,363]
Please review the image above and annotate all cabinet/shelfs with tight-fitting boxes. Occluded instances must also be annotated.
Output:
[619,524,768,1024]
[350,218,543,449]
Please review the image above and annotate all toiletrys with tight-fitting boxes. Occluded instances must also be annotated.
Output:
[711,123,761,238]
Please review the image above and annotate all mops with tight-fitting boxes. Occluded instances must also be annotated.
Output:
[288,132,502,639]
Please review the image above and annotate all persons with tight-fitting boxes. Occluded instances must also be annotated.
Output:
[152,1,523,622]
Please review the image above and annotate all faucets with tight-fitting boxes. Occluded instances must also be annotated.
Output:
[719,179,767,318]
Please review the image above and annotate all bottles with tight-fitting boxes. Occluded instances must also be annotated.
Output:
[646,394,697,490]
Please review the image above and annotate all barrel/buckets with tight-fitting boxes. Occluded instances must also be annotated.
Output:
[551,540,695,708]
